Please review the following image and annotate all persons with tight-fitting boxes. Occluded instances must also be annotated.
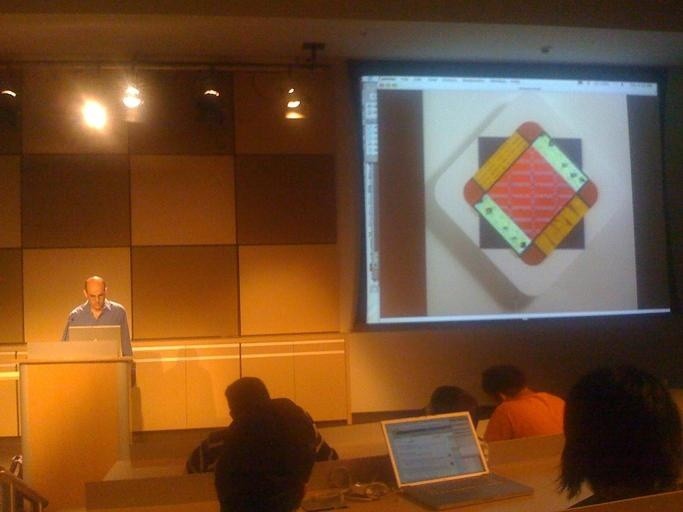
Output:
[417,385,485,446]
[59,275,133,364]
[209,377,337,511]
[183,375,340,475]
[553,363,682,509]
[483,363,566,443]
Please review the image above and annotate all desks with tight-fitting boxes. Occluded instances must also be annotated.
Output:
[85,432,682,512]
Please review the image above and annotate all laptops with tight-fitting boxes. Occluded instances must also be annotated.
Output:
[380,412,535,511]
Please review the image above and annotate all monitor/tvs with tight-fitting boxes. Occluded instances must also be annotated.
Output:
[67,325,121,356]
